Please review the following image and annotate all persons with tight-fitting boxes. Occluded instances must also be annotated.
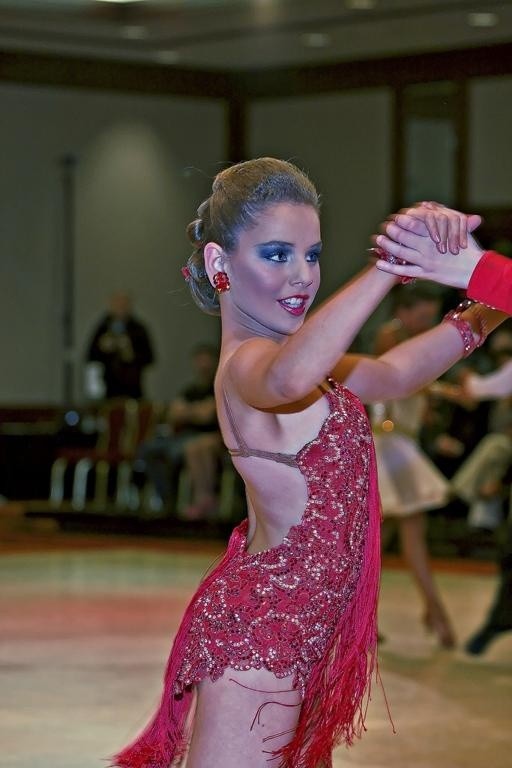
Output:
[165,154,512,766]
[82,289,156,402]
[361,288,512,654]
[371,197,512,315]
[165,342,227,518]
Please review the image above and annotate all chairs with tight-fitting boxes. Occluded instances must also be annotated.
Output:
[48,396,248,523]
